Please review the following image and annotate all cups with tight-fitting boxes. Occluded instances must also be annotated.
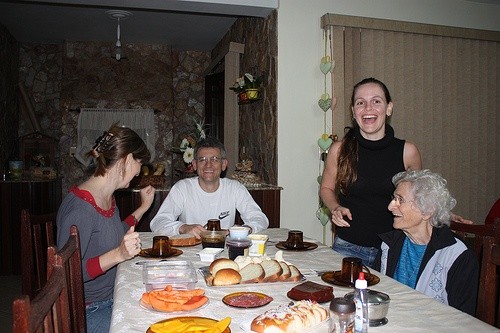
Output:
[342,257,362,282]
[228,227,249,239]
[226,238,252,260]
[208,219,221,231]
[152,236,170,256]
[201,231,227,249]
[286,231,304,248]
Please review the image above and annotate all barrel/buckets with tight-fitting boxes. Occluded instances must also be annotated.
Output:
[8,160,24,178]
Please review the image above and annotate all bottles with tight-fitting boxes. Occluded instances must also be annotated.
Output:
[329,272,369,333]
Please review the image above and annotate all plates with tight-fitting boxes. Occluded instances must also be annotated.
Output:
[198,266,306,289]
[170,239,202,247]
[222,292,273,308]
[275,241,319,252]
[138,248,183,258]
[138,299,210,315]
[321,271,381,288]
[146,316,257,333]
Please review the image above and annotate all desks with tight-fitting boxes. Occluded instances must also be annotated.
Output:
[109,227,500,333]
[126,182,284,232]
[0,176,62,275]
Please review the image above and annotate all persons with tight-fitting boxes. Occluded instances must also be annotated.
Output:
[319,77,473,267]
[371,169,479,317]
[56,120,156,333]
[150,136,269,240]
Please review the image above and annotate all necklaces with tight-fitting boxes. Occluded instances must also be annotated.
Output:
[403,239,426,283]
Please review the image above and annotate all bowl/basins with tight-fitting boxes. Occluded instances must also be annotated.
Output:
[344,288,390,327]
[198,247,224,263]
[142,260,197,292]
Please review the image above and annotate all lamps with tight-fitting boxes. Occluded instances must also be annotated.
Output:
[104,10,134,60]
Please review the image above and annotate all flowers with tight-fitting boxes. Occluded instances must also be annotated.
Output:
[172,113,207,164]
[229,72,266,94]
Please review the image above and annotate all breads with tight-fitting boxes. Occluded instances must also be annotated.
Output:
[210,251,303,287]
[169,233,196,245]
[290,280,334,302]
[250,301,328,333]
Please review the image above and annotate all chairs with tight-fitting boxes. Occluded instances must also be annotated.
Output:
[20,209,57,302]
[450,221,500,327]
[13,224,86,333]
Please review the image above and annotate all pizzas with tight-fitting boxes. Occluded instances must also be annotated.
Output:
[151,287,205,304]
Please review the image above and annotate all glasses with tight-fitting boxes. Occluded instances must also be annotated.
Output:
[391,195,417,205]
[195,156,223,163]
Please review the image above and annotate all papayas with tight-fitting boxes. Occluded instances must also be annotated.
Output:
[150,317,231,333]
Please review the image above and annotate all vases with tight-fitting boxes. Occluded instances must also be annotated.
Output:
[240,89,259,100]
